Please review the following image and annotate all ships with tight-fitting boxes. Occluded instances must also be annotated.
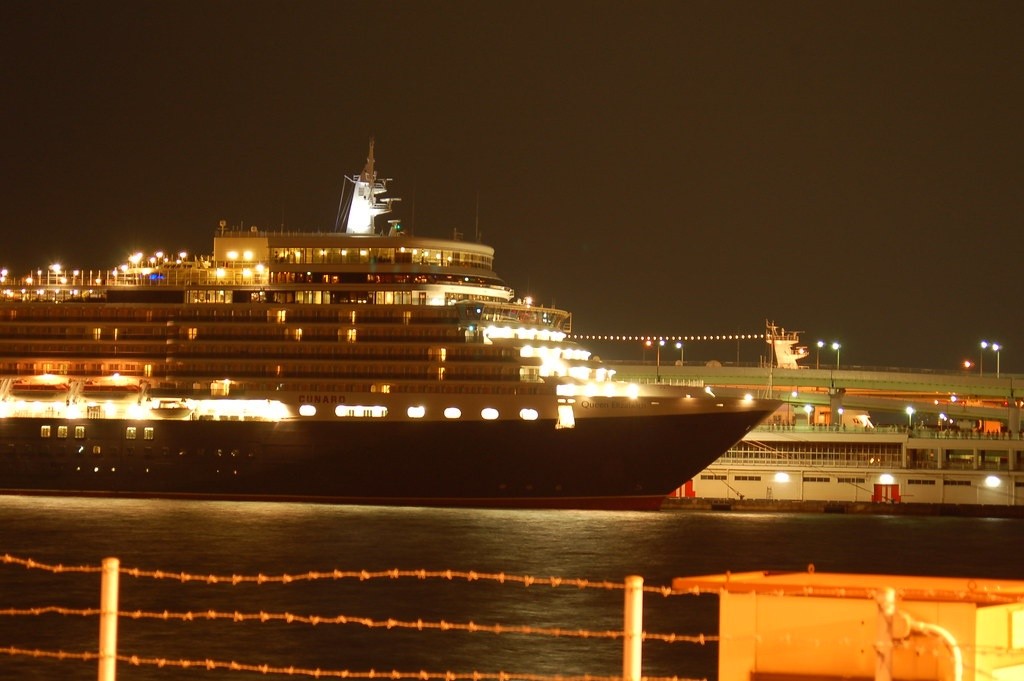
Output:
[0,145,788,510]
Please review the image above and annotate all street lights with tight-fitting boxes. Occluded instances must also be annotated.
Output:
[837,409,843,430]
[832,341,840,370]
[815,341,825,369]
[642,339,651,365]
[991,343,1001,378]
[674,343,684,367]
[979,342,988,376]
[657,339,666,377]
[804,405,812,426]
[906,408,912,427]
